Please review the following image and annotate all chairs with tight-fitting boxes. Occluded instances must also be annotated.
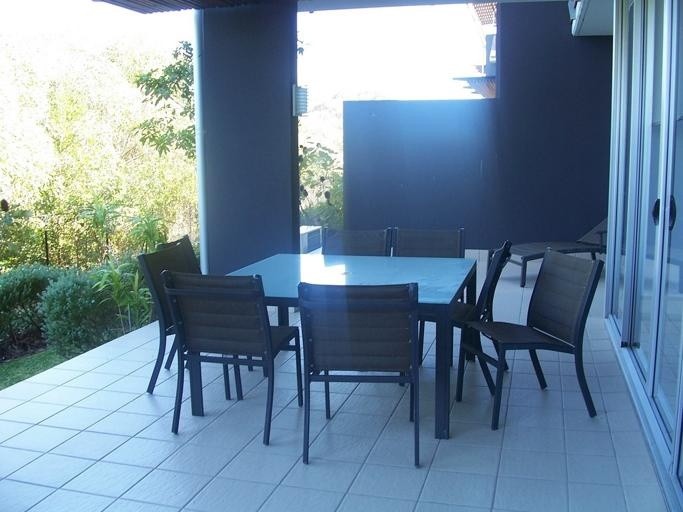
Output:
[486,217,608,288]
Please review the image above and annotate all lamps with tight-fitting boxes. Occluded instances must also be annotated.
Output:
[292,84,308,117]
